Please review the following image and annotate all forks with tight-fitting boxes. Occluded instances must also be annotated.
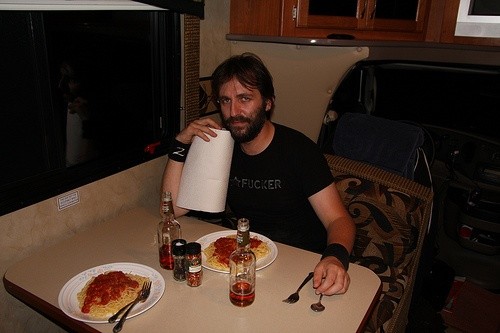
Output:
[113,281,152,333]
[282,272,315,306]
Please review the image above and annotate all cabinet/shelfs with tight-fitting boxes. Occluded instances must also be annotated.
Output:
[218,0,500,54]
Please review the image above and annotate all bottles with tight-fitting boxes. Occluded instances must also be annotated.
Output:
[186,242,202,288]
[229,218,256,307]
[171,238,186,282]
[157,191,181,270]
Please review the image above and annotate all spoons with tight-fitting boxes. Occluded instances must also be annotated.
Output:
[108,288,150,324]
[311,293,326,312]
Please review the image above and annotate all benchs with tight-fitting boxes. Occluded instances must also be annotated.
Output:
[324,151,431,333]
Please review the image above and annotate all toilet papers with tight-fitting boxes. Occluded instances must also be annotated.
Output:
[175,128,235,214]
[64,102,88,165]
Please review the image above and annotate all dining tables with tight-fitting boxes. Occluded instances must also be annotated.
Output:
[4,206,384,333]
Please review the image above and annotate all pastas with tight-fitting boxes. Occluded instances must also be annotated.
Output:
[203,235,271,272]
[77,270,149,317]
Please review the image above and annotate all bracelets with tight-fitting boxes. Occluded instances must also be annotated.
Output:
[168,138,190,162]
[321,243,349,271]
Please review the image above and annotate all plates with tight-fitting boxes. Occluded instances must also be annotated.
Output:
[195,229,279,274]
[57,261,165,321]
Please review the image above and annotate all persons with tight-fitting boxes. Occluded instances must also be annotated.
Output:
[159,52,355,296]
[60,64,122,183]
